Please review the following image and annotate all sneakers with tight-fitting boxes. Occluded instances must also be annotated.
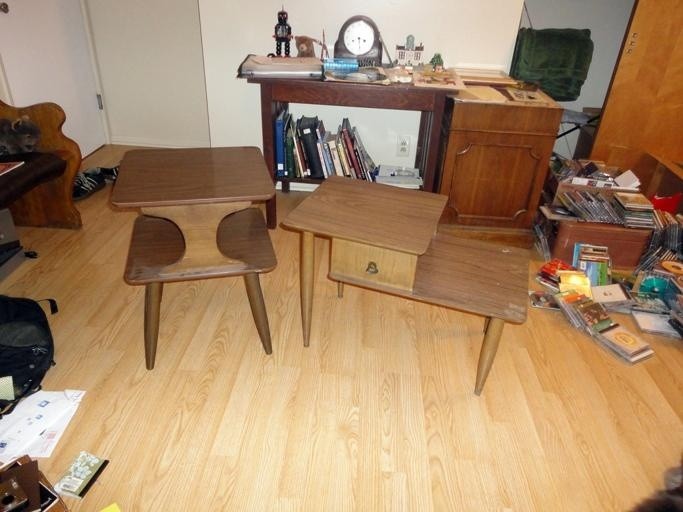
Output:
[101,166,119,180]
[72,168,106,200]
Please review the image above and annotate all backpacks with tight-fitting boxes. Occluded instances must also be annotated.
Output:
[0,294,58,419]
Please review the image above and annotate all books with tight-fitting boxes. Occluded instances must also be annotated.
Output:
[0,459,61,512]
[275,108,424,190]
[53,451,109,498]
[412,63,550,106]
[529,157,683,362]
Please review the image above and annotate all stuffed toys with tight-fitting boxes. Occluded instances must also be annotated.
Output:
[295,34,314,57]
